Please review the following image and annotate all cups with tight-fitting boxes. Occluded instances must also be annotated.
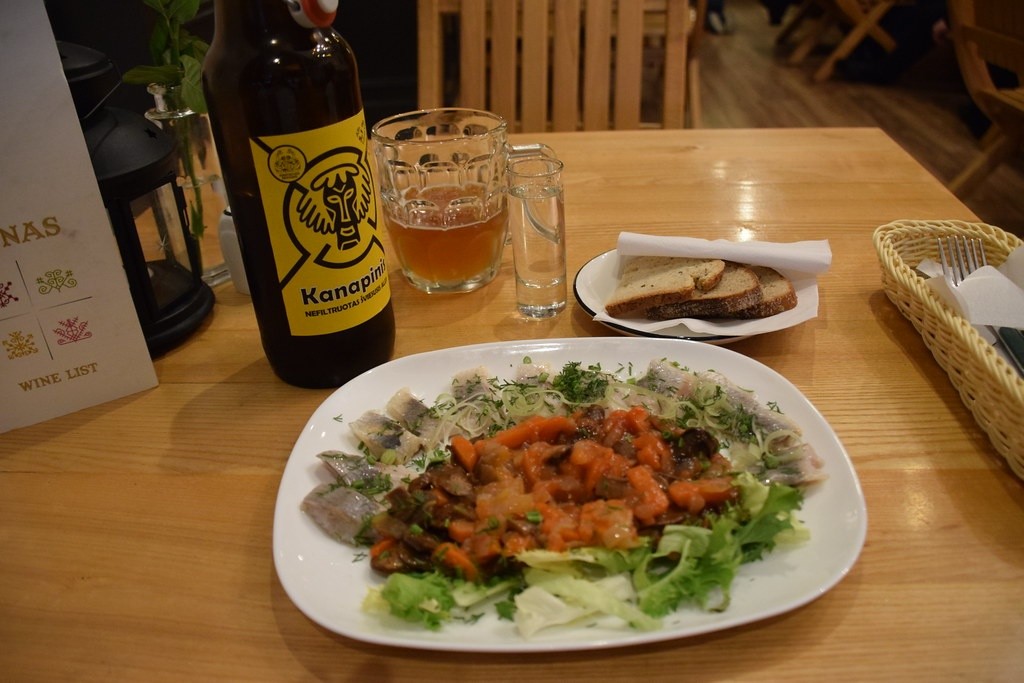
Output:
[373,108,559,293]
[506,156,566,318]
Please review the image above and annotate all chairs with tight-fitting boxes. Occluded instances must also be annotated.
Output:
[417,0,706,141]
[785,1,1023,212]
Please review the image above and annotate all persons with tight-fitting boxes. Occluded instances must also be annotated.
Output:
[690,1,1019,141]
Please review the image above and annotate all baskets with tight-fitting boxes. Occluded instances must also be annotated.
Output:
[872,219,1024,481]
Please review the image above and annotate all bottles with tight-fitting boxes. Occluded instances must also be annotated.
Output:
[202,0,396,388]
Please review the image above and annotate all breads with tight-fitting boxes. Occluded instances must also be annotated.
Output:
[645,261,764,319]
[728,267,797,319]
[604,254,725,318]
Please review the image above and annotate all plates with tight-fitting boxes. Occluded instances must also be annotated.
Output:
[572,247,752,345]
[272,337,867,653]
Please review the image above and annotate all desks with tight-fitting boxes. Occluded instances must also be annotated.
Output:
[0,127,1024,683]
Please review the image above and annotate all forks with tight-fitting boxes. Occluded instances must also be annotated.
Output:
[937,236,1024,377]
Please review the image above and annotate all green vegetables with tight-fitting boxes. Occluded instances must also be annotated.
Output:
[316,355,811,632]
